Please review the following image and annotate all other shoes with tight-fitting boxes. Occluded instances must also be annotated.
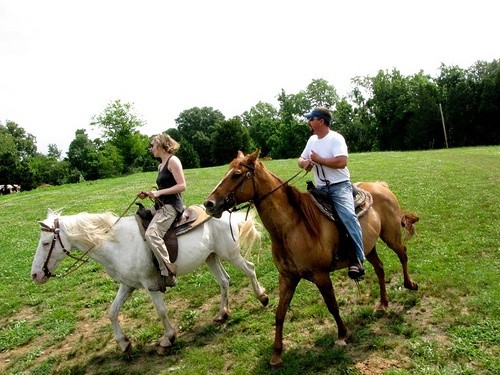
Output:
[350,261,363,273]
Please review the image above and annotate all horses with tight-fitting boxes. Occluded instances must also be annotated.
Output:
[202,148,418,365]
[29,208,270,358]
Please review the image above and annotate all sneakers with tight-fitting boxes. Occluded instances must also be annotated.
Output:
[148,274,177,291]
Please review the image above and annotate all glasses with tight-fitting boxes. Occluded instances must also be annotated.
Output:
[150,142,156,148]
[309,118,320,121]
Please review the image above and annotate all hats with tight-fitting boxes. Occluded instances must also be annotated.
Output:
[303,107,331,121]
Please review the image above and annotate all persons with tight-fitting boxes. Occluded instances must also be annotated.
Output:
[137,134,187,291]
[299,108,366,277]
